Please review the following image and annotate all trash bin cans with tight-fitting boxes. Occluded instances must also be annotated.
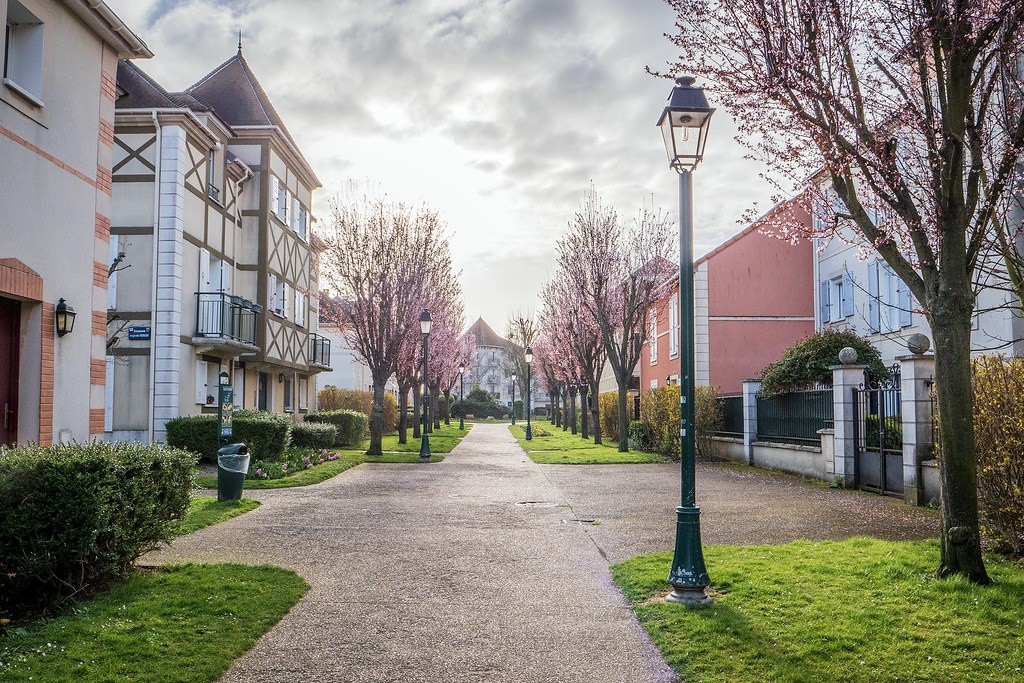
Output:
[217,442,250,501]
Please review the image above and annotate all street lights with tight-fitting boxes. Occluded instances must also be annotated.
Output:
[511,370,517,425]
[656,77,718,608]
[458,361,465,430]
[523,346,534,440]
[418,309,434,459]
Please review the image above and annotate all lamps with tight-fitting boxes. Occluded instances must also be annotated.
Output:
[279,372,286,383]
[665,375,670,386]
[55,297,77,338]
